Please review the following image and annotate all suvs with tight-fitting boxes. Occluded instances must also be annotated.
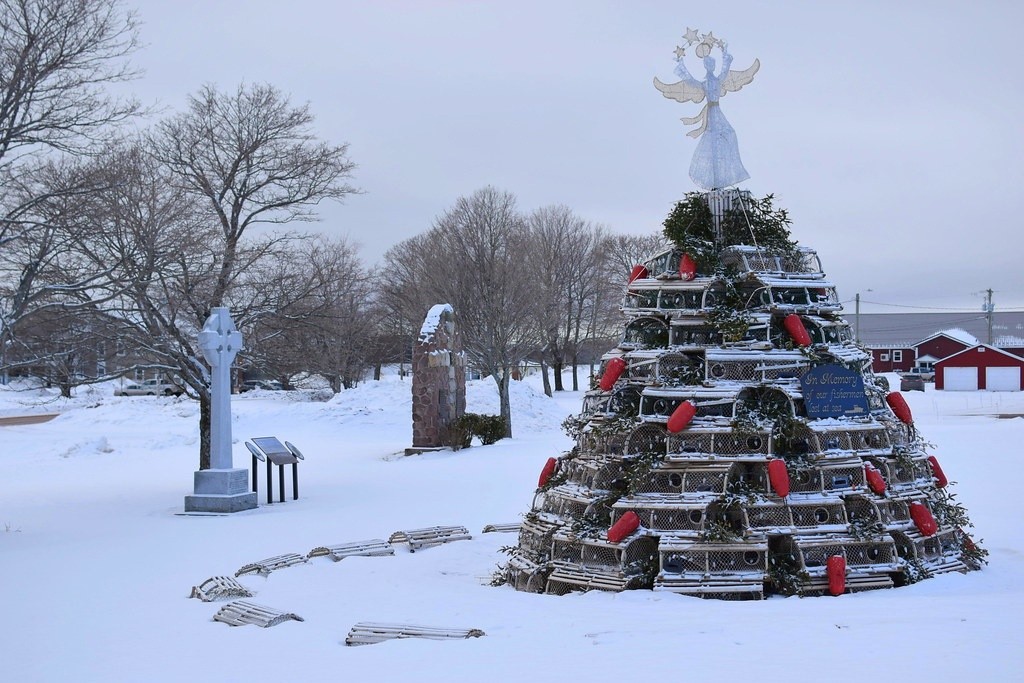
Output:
[900,374,924,392]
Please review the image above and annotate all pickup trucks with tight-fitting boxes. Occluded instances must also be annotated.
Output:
[897,367,935,382]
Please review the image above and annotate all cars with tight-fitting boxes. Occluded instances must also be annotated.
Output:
[875,376,889,391]
